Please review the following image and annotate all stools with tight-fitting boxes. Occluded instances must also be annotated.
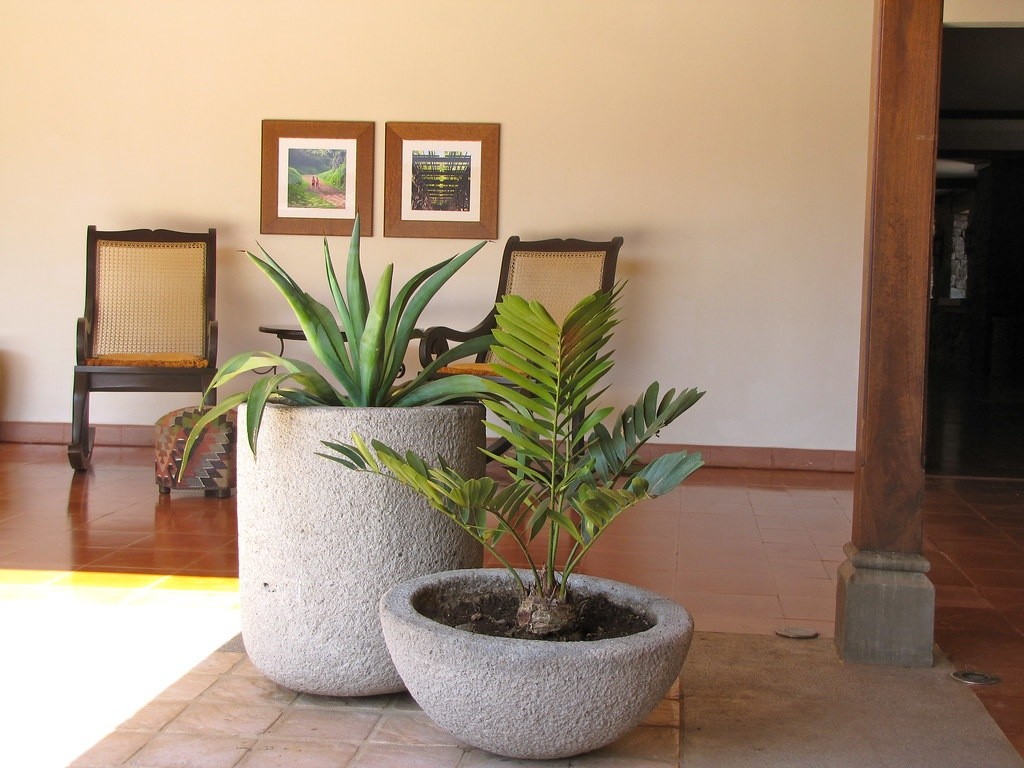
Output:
[154,404,237,499]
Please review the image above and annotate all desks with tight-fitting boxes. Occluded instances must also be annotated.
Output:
[252,324,425,391]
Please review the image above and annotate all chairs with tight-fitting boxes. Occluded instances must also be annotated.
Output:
[419,235,627,482]
[67,224,219,471]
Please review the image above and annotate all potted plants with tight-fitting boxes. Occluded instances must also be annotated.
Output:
[310,278,708,762]
[174,210,586,698]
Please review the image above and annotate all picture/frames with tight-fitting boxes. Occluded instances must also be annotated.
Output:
[382,123,499,240]
[260,119,373,237]
